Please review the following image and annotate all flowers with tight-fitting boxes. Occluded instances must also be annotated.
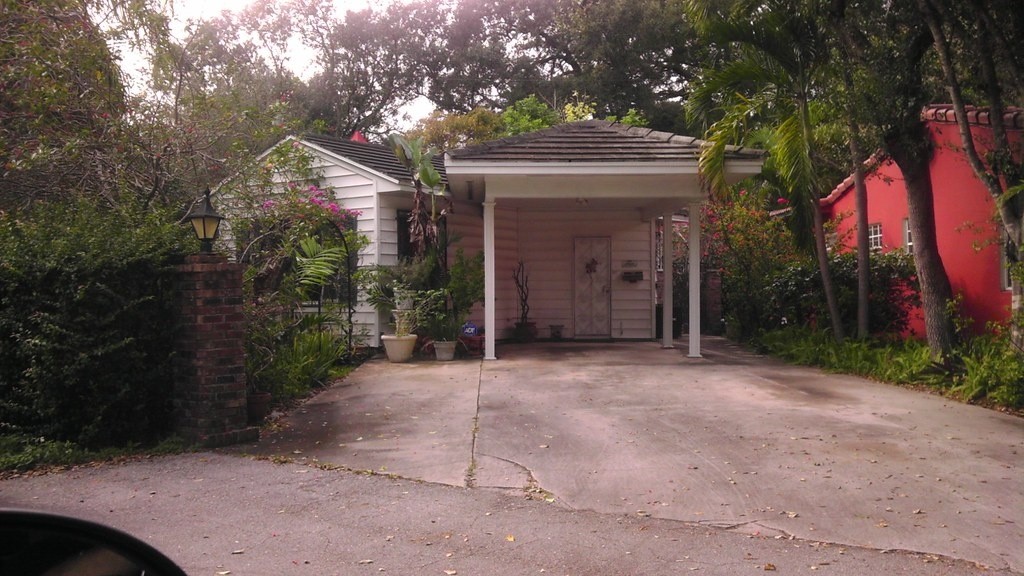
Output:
[369,256,434,288]
[371,287,445,335]
[426,310,465,341]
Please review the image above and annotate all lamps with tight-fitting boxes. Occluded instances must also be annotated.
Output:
[190,183,224,255]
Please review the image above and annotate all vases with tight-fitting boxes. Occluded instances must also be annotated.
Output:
[380,333,417,362]
[433,341,457,360]
[393,289,417,310]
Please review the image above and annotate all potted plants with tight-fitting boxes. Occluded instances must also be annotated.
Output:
[512,258,536,342]
